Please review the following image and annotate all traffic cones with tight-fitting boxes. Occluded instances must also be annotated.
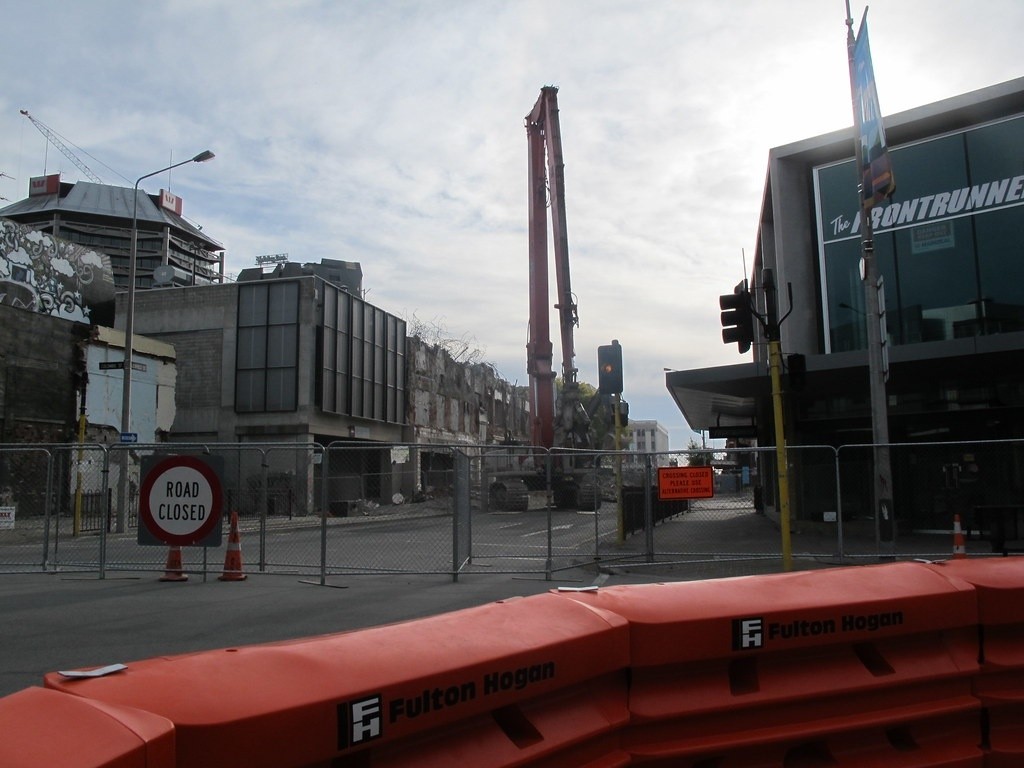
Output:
[217,511,249,581]
[156,546,189,583]
[952,514,968,559]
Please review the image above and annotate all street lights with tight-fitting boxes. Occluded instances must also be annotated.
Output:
[117,151,215,533]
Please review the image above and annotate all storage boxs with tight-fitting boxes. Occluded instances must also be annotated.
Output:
[328,500,363,517]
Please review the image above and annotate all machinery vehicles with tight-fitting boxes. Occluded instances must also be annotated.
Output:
[490,84,628,513]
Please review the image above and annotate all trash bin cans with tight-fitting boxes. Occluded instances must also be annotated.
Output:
[754,485,763,512]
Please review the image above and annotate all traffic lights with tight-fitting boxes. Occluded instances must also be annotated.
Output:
[720,278,753,353]
[598,345,624,395]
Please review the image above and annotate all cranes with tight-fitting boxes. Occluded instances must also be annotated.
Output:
[19,109,136,188]
